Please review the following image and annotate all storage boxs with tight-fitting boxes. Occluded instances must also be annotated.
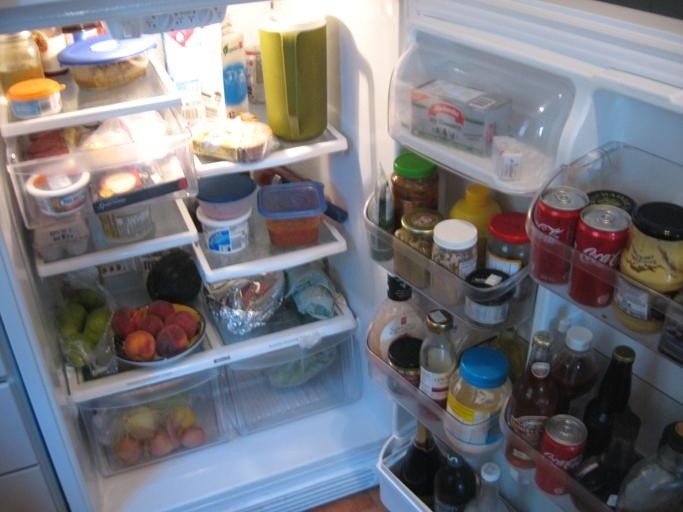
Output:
[4,107,198,230]
[73,283,223,477]
[207,284,362,436]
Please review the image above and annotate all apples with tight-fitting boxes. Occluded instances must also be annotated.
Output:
[112,407,205,465]
[59,288,110,368]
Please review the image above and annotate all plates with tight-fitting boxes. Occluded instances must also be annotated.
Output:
[111,301,206,369]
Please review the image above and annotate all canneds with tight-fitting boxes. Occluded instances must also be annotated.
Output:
[531,186,590,284]
[568,203,632,307]
[535,414,589,495]
[391,153,532,306]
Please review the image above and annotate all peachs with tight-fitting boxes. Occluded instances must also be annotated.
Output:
[112,299,198,362]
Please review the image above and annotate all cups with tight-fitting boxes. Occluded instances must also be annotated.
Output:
[0,29,46,98]
[440,346,514,456]
[258,15,332,144]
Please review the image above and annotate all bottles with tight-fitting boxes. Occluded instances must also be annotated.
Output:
[368,273,427,379]
[398,420,442,511]
[549,325,602,420]
[216,16,251,119]
[417,308,458,420]
[463,460,509,512]
[612,419,683,512]
[432,447,477,512]
[570,343,642,511]
[501,330,559,468]
